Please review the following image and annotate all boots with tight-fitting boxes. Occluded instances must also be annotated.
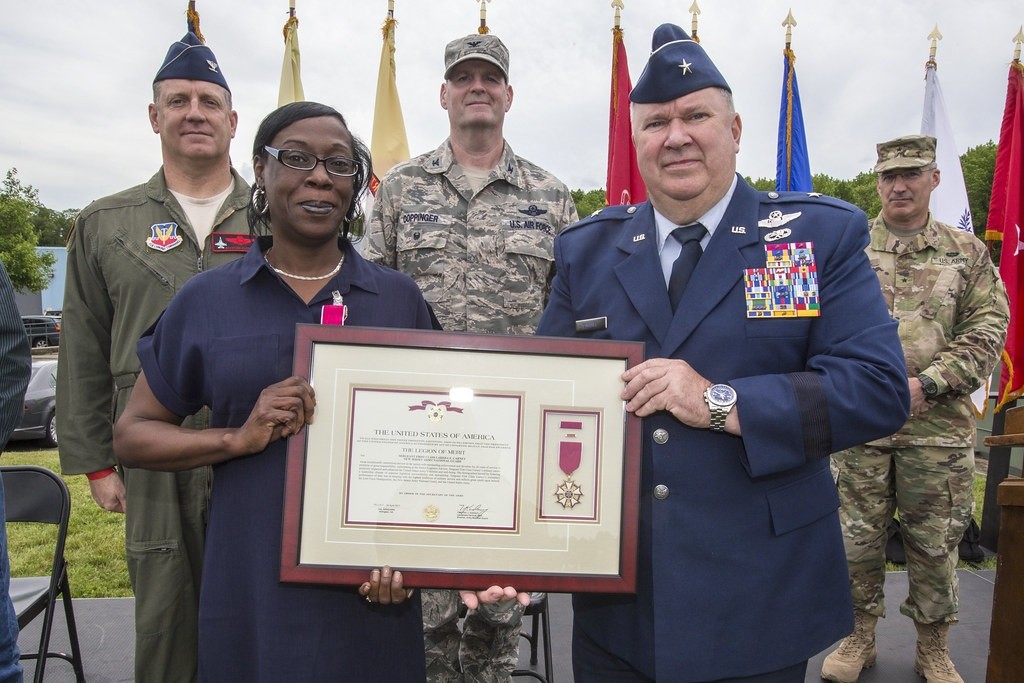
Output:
[820,612,877,683]
[913,618,964,683]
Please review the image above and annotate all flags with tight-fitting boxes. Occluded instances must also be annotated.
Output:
[186,15,1023,417]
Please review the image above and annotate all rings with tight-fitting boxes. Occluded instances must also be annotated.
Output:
[365,595,373,603]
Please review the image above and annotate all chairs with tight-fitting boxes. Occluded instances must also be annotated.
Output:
[511,593,554,682]
[0,465,85,683]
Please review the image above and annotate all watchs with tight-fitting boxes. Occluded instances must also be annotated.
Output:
[703,382,737,434]
[916,374,938,400]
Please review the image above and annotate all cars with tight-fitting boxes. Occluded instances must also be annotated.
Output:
[23,309,63,349]
[7,360,58,447]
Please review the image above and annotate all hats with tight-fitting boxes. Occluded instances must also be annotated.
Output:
[153,26,232,95]
[875,135,938,172]
[444,34,509,86]
[628,25,732,104]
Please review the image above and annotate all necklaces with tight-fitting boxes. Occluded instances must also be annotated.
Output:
[264,247,345,280]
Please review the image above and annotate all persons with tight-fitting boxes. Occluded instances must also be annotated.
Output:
[109,101,444,682]
[819,135,1010,683]
[56,31,271,682]
[452,22,911,683]
[359,33,582,683]
[0,264,32,683]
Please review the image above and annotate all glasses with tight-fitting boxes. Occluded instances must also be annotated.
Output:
[262,144,362,177]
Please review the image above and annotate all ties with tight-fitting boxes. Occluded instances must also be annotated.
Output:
[669,225,709,316]
